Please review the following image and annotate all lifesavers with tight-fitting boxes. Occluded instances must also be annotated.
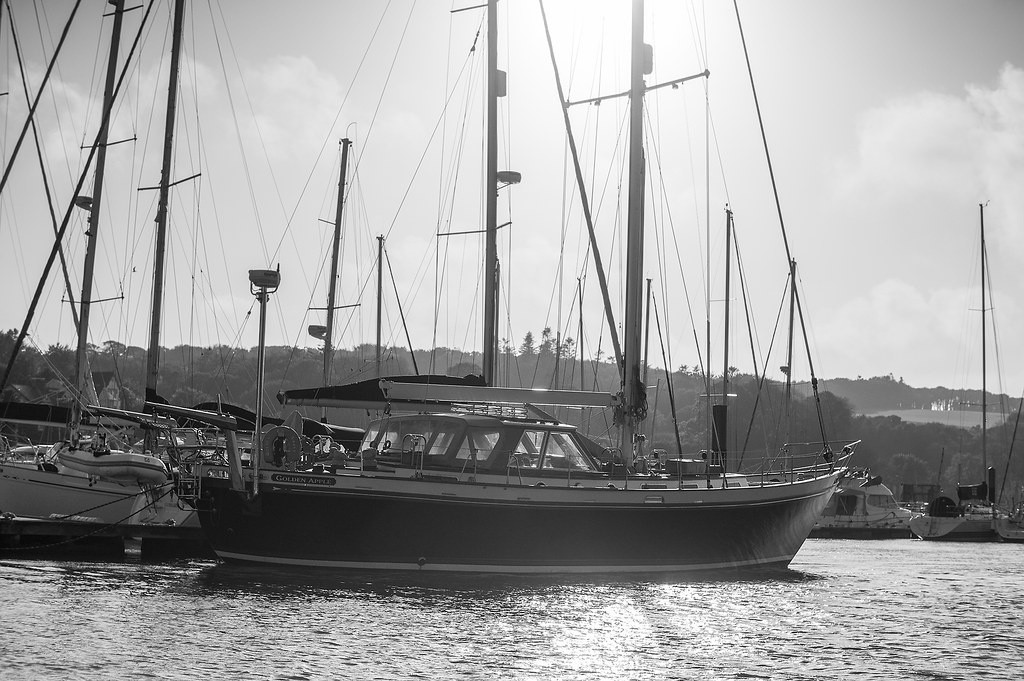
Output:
[262,426,302,464]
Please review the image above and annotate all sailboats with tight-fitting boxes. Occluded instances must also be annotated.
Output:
[0,2,1024,585]
